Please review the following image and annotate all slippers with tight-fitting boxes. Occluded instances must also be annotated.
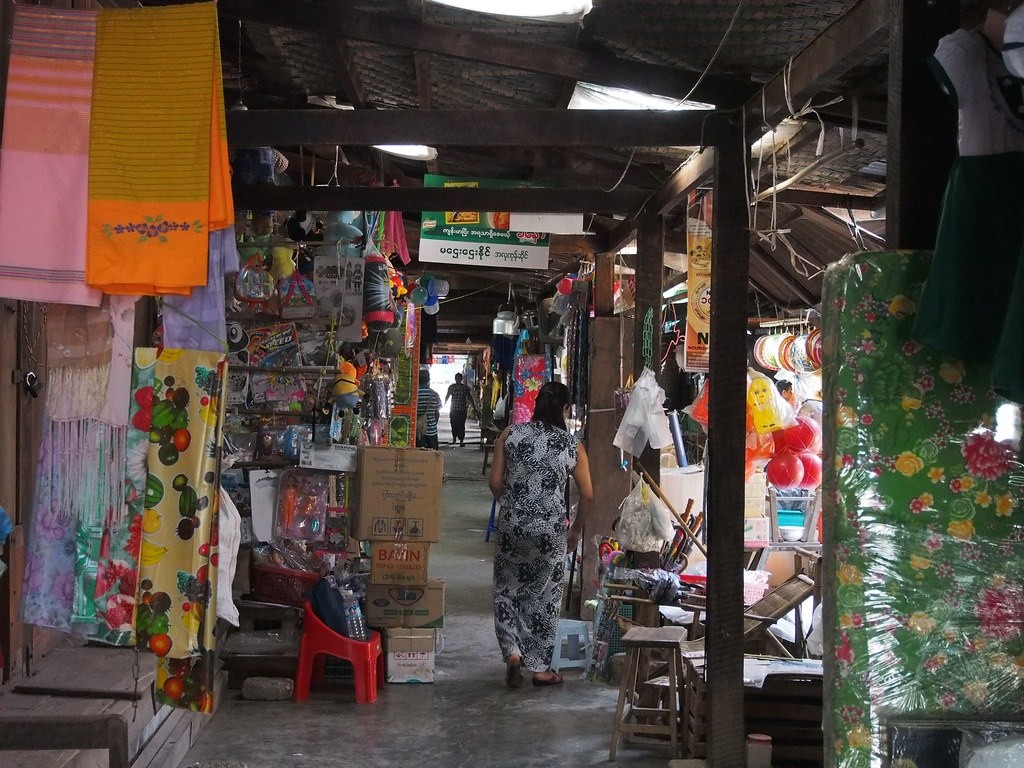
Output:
[505,660,524,687]
[532,669,564,686]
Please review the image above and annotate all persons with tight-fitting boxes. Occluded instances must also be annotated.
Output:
[445,373,476,447]
[776,379,793,400]
[487,382,594,688]
[282,475,297,534]
[416,370,447,483]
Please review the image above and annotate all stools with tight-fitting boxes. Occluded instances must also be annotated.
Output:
[608,625,688,763]
[290,597,385,704]
[480,443,495,477]
[484,497,499,542]
[549,619,594,676]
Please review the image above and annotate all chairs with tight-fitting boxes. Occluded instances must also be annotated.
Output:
[626,573,818,700]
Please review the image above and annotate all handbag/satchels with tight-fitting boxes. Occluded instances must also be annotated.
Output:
[417,413,427,439]
[303,577,348,635]
[493,397,505,420]
[618,472,675,553]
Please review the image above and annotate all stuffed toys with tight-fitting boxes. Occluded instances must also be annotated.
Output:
[251,210,408,418]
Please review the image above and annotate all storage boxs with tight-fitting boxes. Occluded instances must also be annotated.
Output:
[380,626,439,685]
[350,445,444,544]
[365,579,444,628]
[369,539,433,586]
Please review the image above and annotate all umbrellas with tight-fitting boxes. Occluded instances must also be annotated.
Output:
[586,498,703,677]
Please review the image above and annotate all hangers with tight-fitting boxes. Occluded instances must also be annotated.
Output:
[151,296,231,356]
[658,299,686,358]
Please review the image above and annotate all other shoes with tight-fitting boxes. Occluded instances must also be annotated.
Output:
[460,443,465,448]
